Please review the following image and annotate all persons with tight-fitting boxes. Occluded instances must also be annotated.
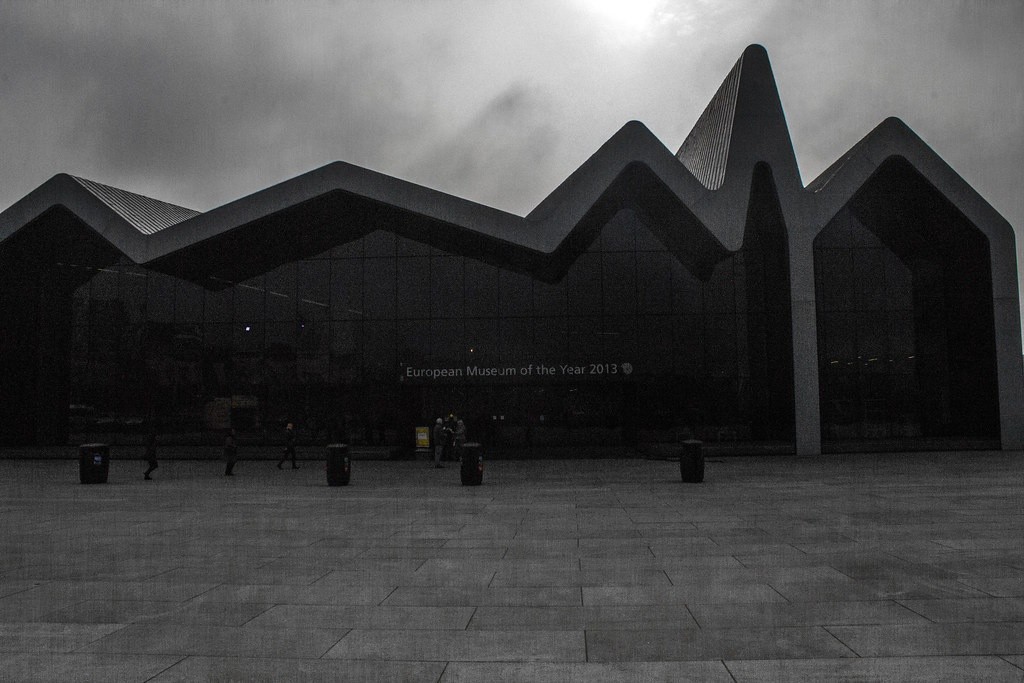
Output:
[277,423,301,470]
[224,428,239,476]
[433,417,467,469]
[142,431,162,480]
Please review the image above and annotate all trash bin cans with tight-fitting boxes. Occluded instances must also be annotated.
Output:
[460,443,483,485]
[680,440,705,483]
[80,444,109,484]
[327,443,350,485]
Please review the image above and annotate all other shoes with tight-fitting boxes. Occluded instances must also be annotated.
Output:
[292,464,298,469]
[277,464,283,470]
[435,463,442,468]
[224,473,234,475]
[144,471,153,480]
